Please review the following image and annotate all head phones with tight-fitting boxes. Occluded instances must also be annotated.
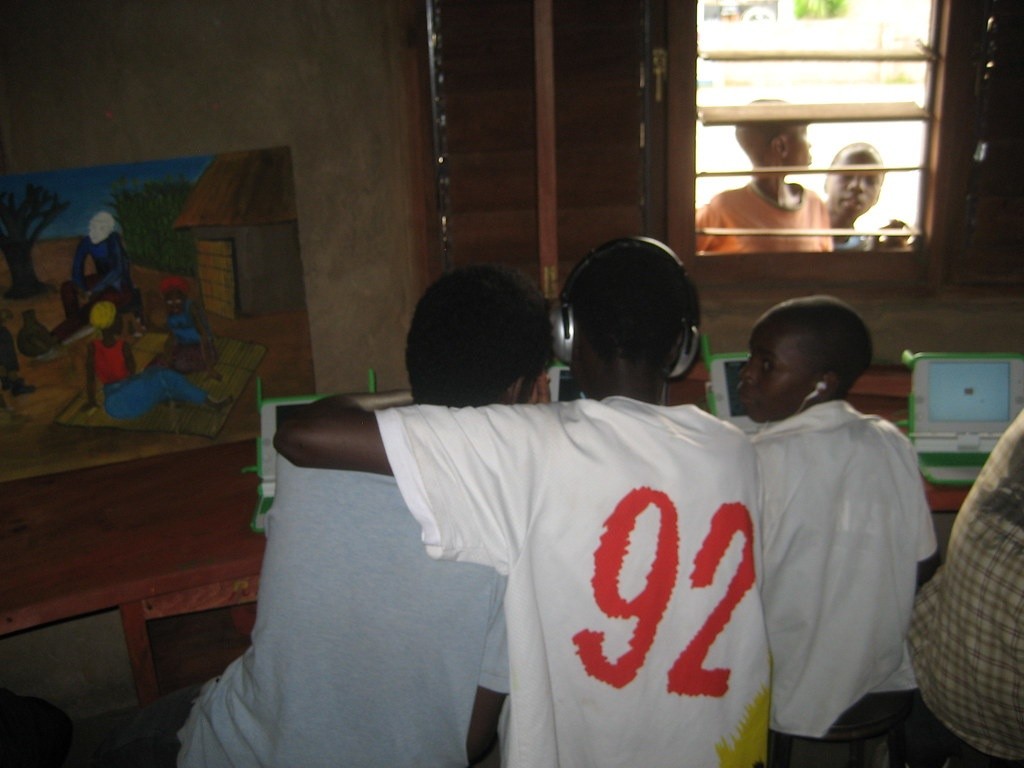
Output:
[546,235,702,385]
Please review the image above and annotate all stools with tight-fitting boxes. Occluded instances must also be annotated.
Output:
[769,691,913,768]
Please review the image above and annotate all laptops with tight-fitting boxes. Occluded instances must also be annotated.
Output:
[699,333,767,435]
[895,349,1024,485]
[545,359,586,403]
[241,367,375,533]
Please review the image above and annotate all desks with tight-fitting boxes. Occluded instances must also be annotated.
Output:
[666,363,970,513]
[0,439,264,635]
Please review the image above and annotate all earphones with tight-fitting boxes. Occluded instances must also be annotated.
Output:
[805,382,827,402]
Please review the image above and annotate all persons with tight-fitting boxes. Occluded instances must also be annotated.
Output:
[693,96,834,253]
[86,266,554,768]
[272,235,771,768]
[739,294,938,768]
[822,140,912,249]
[905,405,1024,768]
